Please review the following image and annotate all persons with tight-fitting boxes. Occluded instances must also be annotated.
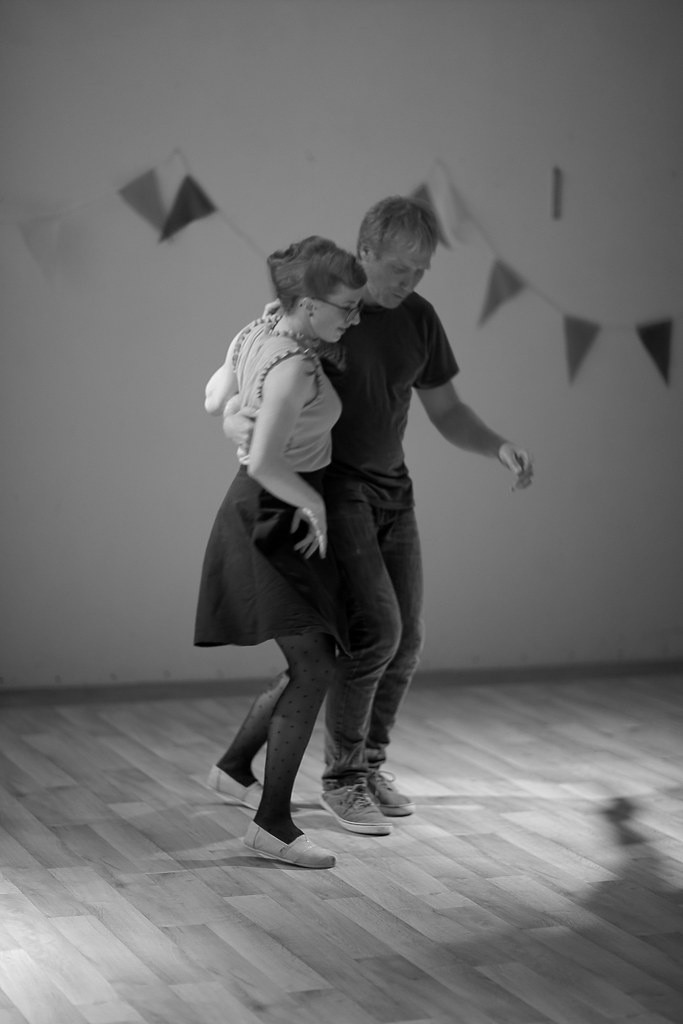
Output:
[193,196,534,869]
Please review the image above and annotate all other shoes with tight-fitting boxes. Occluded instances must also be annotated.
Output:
[206,764,264,811]
[242,820,337,868]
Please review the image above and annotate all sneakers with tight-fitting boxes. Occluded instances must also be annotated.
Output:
[366,766,416,815]
[318,783,393,834]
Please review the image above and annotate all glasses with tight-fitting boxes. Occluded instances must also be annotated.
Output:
[299,296,365,323]
